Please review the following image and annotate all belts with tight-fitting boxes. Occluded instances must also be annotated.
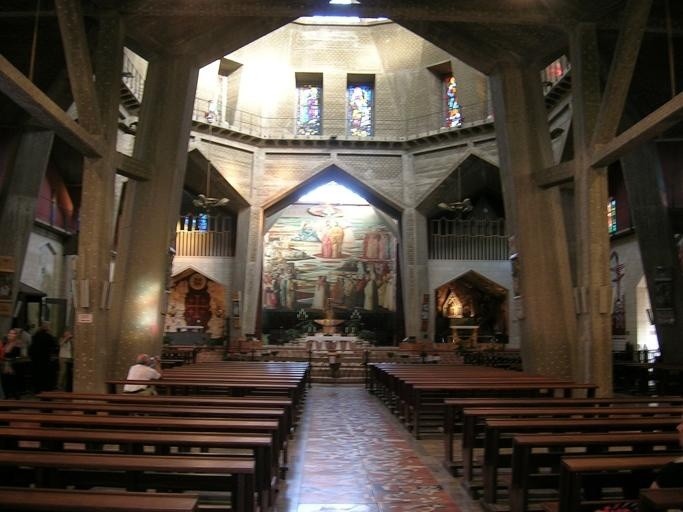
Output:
[123,389,143,394]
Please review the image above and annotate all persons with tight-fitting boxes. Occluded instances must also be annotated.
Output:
[435,310,451,343]
[258,215,398,313]
[0,321,74,413]
[123,353,163,416]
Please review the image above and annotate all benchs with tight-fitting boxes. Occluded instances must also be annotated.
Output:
[366,361,683,512]
[0,361,311,512]
